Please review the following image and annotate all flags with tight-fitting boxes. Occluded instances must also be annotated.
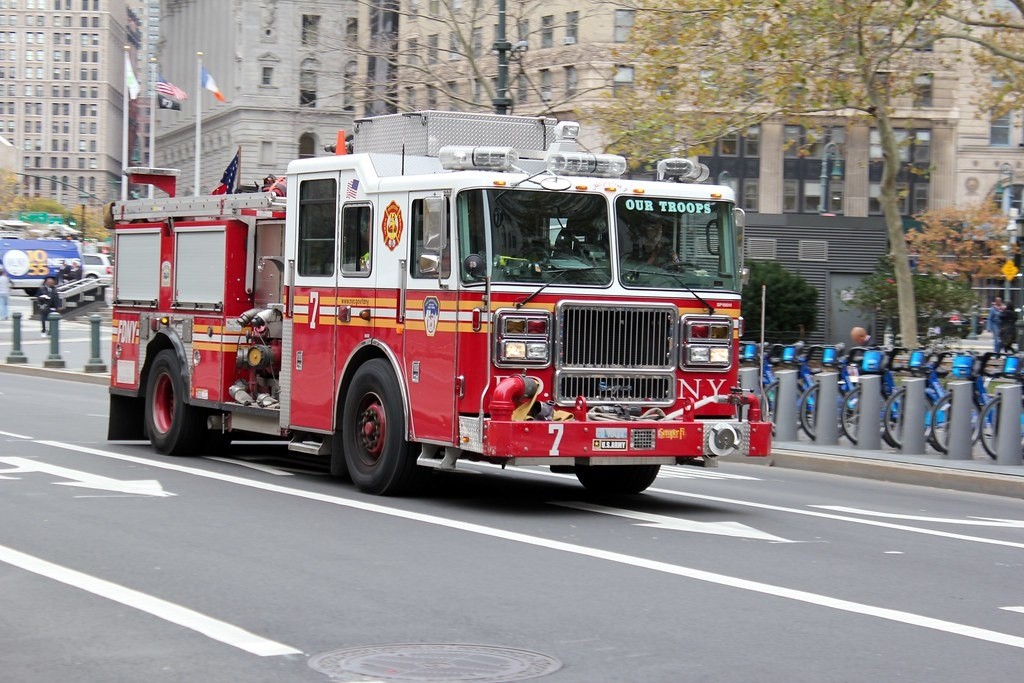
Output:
[154,64,186,101]
[210,146,240,193]
[125,53,139,102]
[200,64,227,102]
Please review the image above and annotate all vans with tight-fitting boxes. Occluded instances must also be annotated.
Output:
[0,239,82,296]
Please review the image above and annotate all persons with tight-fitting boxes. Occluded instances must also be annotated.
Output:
[37,259,79,334]
[633,218,680,269]
[989,295,1019,359]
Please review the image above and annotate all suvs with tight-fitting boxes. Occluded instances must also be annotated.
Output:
[83,253,114,278]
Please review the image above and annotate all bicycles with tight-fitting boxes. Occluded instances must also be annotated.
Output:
[738,341,1024,459]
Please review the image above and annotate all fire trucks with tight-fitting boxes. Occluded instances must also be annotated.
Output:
[103,112,774,497]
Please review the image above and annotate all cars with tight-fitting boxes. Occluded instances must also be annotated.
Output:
[944,314,971,339]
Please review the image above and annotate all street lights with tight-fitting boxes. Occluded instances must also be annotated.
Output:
[817,143,843,213]
